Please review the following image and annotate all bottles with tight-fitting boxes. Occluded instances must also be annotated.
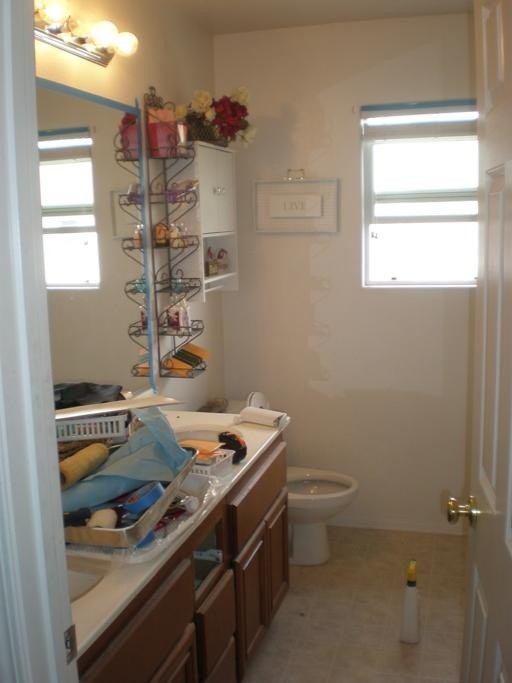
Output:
[163,294,191,333]
[133,223,145,248]
[155,222,187,247]
[139,296,149,331]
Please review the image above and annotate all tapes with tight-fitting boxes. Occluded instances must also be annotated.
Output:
[123,481,164,512]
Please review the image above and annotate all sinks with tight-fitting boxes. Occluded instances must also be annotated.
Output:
[67,552,111,604]
[174,425,242,442]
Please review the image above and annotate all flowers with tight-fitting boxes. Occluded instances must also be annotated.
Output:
[174,85,257,144]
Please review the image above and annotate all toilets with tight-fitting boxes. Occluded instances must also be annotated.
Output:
[214,393,358,567]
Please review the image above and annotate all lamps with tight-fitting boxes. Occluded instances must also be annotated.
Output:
[31,1,139,69]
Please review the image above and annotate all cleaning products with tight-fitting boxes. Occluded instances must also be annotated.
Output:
[399,560,420,643]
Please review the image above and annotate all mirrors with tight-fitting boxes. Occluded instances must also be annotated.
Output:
[35,77,157,414]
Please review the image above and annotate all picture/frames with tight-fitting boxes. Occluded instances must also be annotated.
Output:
[250,176,341,235]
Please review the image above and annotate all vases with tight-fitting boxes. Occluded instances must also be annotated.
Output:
[189,124,231,146]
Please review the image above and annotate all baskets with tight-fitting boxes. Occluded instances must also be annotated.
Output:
[187,446,237,479]
[55,414,129,445]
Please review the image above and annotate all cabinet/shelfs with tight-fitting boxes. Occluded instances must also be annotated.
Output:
[113,97,152,379]
[171,140,242,302]
[141,85,210,383]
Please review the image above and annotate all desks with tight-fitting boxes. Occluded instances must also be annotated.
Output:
[53,408,291,681]
